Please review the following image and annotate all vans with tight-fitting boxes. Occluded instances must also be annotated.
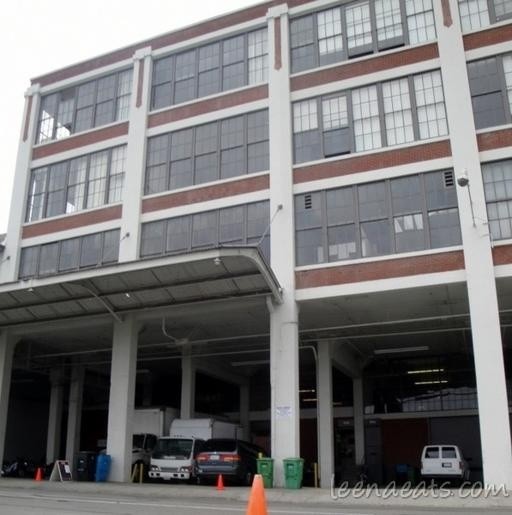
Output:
[419,443,473,486]
[195,437,266,488]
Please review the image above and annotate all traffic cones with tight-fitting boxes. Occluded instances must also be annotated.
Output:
[216,473,228,491]
[244,472,272,515]
[34,466,44,482]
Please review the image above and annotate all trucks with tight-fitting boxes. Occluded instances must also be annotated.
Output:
[130,405,228,480]
[149,416,245,483]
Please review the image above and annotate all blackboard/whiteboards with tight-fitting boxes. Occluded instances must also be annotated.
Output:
[57,460,72,479]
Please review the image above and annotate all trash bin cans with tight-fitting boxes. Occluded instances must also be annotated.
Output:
[256,458,273,488]
[283,458,305,489]
